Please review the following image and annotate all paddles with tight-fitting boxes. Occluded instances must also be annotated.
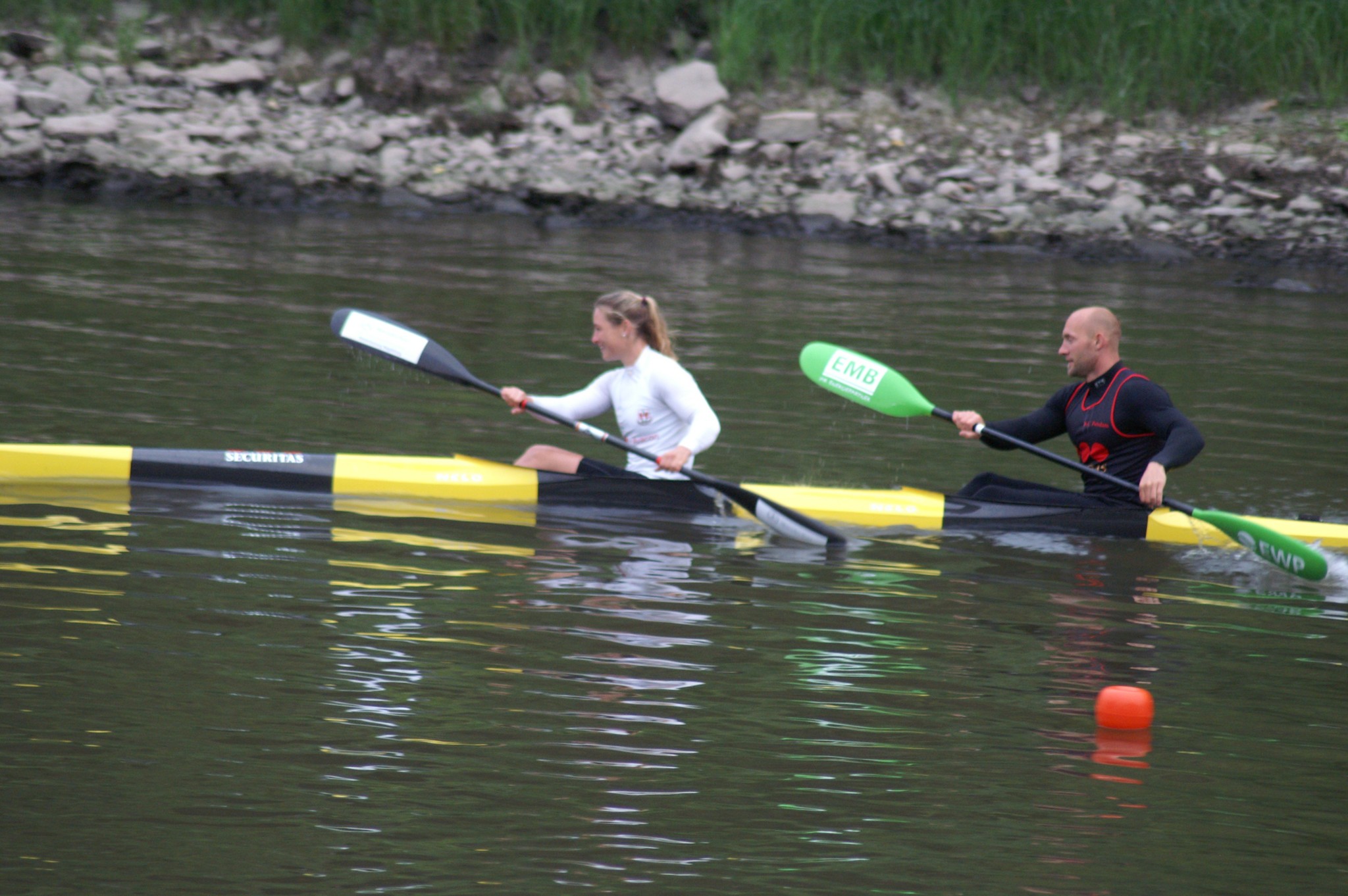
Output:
[329,304,846,545]
[799,338,1331,583]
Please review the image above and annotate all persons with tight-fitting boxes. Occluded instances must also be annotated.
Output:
[953,307,1206,514]
[496,289,722,484]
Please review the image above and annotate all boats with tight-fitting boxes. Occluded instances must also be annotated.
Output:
[0,445,1348,555]
